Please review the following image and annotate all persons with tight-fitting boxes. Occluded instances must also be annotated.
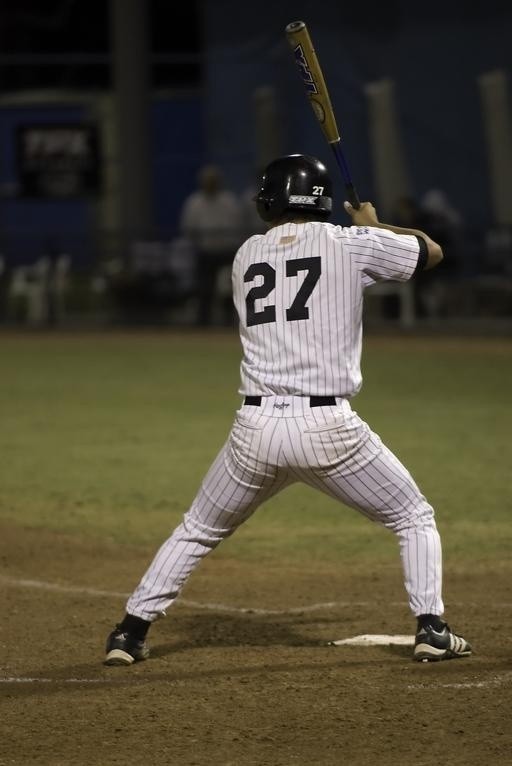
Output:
[103,153,475,665]
[0,158,512,325]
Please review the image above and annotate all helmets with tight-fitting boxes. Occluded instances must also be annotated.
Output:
[251,153,333,222]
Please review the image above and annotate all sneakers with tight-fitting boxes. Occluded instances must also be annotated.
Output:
[414,619,472,663]
[105,623,150,665]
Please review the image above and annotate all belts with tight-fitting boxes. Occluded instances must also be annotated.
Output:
[244,396,336,407]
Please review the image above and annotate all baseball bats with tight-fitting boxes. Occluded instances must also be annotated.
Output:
[283,21,361,211]
